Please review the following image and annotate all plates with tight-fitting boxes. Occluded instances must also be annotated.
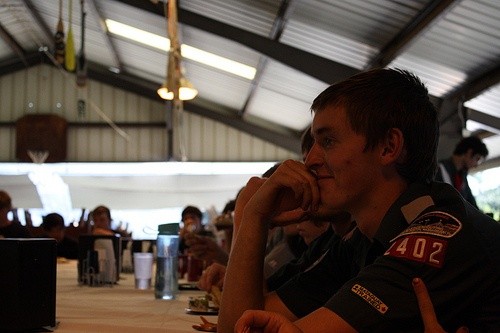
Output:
[185,307,219,315]
[178,284,201,291]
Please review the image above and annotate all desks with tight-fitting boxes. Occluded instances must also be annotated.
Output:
[55,259,219,333]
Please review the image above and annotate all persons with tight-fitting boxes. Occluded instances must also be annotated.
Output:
[179,124,353,333]
[0,191,133,253]
[217,67,500,333]
[437,136,489,209]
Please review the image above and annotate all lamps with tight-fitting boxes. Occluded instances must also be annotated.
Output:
[157,53,198,101]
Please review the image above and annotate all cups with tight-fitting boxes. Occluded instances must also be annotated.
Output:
[154,223,181,301]
[133,253,153,289]
